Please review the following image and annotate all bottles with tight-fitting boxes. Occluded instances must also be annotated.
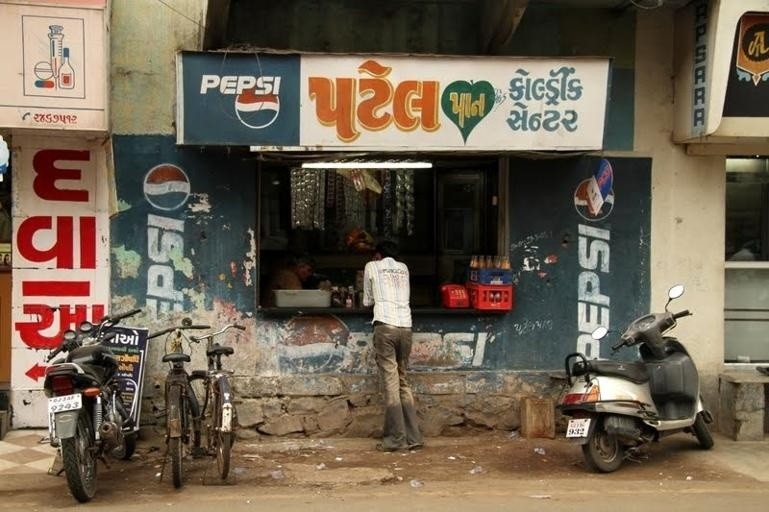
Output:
[332,285,356,308]
[468,255,511,270]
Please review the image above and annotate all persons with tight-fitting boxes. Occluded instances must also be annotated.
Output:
[267,256,316,303]
[362,237,425,452]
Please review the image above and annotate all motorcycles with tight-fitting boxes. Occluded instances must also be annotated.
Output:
[555,284,716,473]
[39,308,142,503]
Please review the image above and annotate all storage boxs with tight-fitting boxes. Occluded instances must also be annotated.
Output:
[272,288,332,308]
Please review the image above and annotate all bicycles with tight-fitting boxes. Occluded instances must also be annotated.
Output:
[145,318,247,488]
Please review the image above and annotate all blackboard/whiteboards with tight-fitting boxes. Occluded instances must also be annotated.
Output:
[82,323,150,429]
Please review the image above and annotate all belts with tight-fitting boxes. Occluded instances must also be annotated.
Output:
[373,319,389,326]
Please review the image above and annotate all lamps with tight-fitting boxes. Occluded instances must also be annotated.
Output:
[302,161,432,169]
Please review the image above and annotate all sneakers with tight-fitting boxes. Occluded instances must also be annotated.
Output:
[375,438,424,454]
